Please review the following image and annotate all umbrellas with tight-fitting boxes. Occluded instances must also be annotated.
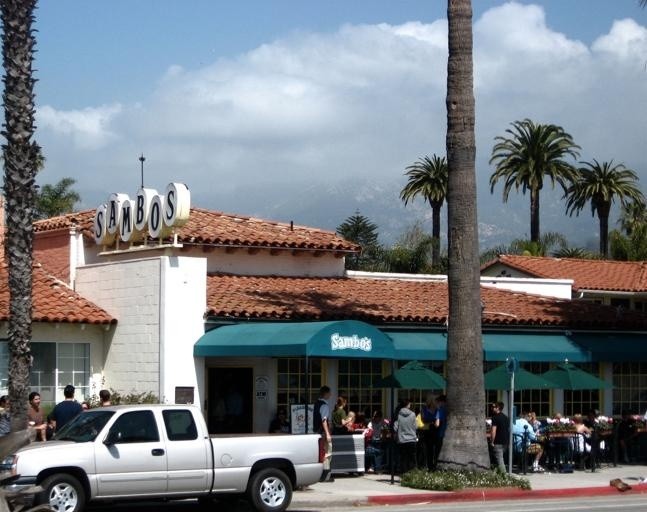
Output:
[371,361,447,390]
[485,362,560,393]
[540,358,609,392]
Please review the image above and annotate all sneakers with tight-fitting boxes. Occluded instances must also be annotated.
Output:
[530,466,545,473]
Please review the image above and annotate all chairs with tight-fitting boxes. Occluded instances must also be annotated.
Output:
[513,431,647,476]
[348,428,444,472]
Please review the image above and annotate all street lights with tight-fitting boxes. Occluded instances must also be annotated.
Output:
[504,356,519,473]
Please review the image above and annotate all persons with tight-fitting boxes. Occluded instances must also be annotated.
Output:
[313,384,334,481]
[0,384,112,475]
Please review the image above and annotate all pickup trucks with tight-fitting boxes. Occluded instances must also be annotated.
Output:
[0,404,329,511]
